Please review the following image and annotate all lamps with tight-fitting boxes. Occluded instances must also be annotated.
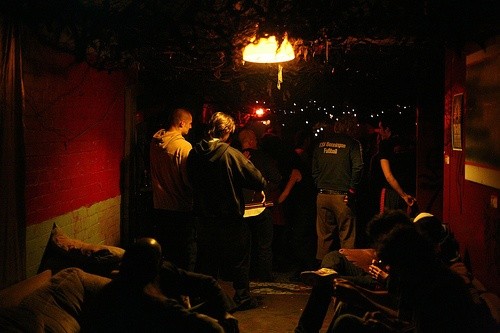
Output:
[243,30,295,63]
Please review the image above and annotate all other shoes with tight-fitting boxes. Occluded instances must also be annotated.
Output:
[233,291,263,307]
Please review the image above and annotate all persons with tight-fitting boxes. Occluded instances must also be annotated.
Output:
[81,106,495,333]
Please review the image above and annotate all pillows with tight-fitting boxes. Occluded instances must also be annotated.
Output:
[38,223,120,275]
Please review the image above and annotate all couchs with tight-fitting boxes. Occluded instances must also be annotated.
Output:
[1,264,119,332]
[340,248,500,333]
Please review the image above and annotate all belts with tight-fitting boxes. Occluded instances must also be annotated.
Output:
[318,189,348,195]
